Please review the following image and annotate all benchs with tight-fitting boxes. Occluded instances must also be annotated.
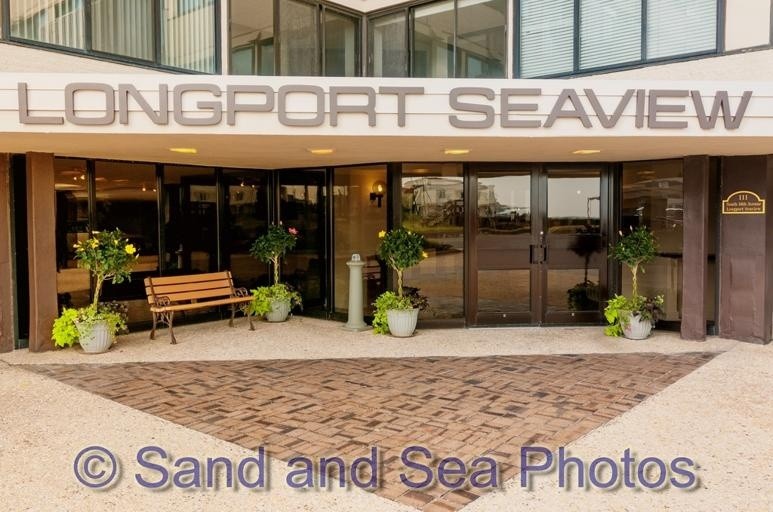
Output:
[141,269,258,346]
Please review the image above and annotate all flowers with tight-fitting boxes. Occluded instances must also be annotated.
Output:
[602,224,665,337]
[49,226,141,347]
[368,228,429,338]
[240,223,305,319]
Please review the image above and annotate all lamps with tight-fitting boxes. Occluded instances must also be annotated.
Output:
[370,180,387,208]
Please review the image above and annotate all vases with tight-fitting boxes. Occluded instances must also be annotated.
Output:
[261,298,292,322]
[383,308,418,339]
[617,308,656,339]
[73,316,118,354]
